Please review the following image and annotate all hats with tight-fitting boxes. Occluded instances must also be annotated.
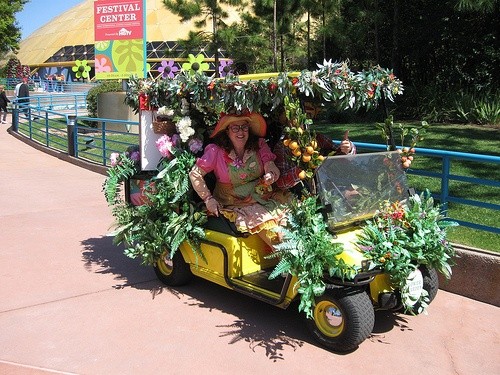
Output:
[208,108,267,137]
[279,101,315,126]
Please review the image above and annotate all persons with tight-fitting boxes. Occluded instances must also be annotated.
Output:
[32,72,42,91]
[44,72,66,93]
[187,112,300,259]
[272,101,359,200]
[13,77,31,119]
[0,85,11,124]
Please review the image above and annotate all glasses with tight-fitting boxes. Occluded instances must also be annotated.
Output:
[228,124,248,133]
